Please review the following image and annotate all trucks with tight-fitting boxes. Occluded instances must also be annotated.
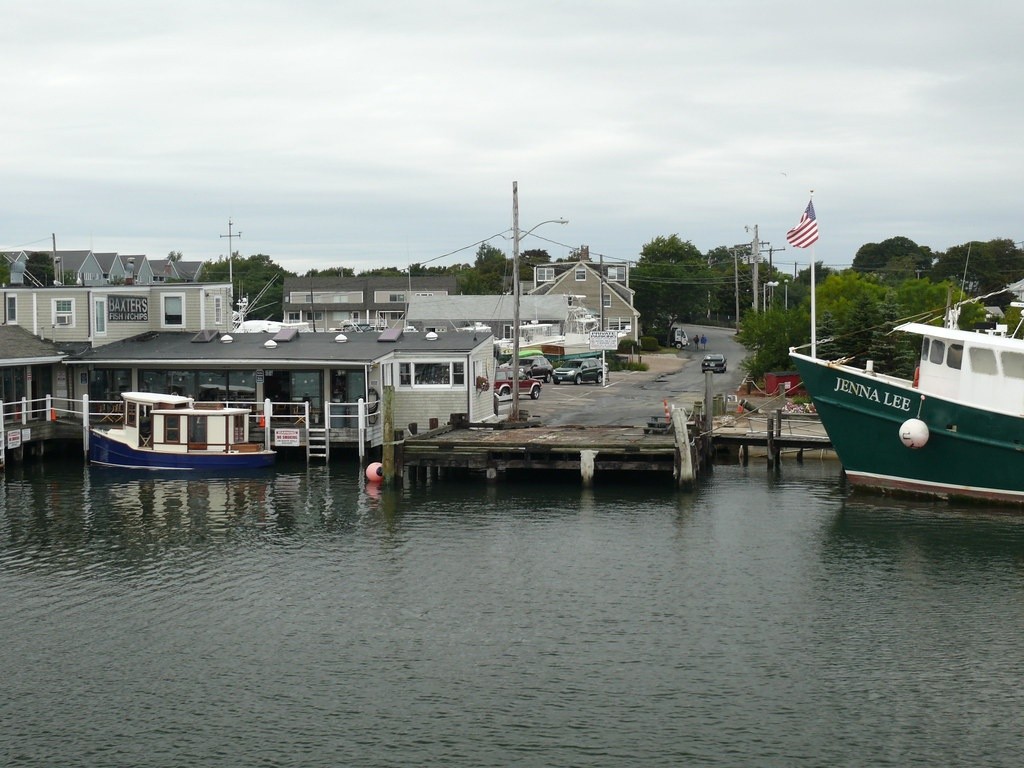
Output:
[664,327,690,350]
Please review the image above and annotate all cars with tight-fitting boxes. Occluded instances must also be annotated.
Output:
[494,368,542,400]
[701,353,726,374]
[499,356,554,384]
[552,358,603,385]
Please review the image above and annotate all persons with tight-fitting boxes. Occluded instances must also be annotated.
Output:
[693,334,707,352]
[139,410,151,446]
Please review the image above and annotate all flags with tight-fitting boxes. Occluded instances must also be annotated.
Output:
[786,199,818,249]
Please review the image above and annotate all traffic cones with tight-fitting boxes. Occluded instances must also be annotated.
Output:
[738,405,743,413]
[664,400,670,421]
[52,409,56,420]
[16,407,20,419]
[260,413,265,426]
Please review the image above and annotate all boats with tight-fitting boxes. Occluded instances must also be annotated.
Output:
[89,391,278,475]
[449,314,628,362]
[788,189,1024,505]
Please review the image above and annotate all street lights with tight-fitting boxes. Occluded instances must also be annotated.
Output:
[764,281,779,312]
[512,219,569,419]
[784,279,789,310]
